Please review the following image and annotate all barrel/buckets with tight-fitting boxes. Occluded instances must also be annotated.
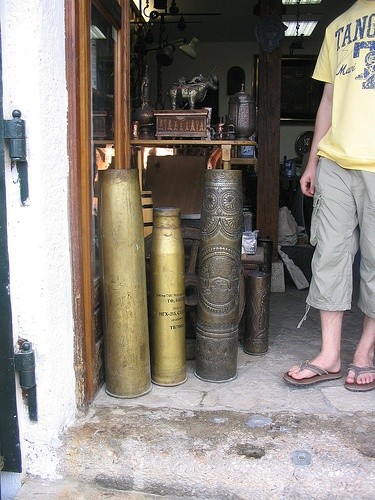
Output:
[241,146,254,157]
[284,159,296,177]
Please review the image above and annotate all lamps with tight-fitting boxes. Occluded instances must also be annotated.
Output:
[288,0,305,49]
[142,44,176,66]
[141,29,202,61]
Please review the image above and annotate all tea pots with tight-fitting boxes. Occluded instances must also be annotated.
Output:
[133,64,155,139]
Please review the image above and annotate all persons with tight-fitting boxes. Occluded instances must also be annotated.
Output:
[283,1,374,392]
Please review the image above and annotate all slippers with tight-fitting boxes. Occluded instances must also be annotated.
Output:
[283,360,342,386]
[344,363,375,392]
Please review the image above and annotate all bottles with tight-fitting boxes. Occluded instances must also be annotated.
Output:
[228,83,257,141]
